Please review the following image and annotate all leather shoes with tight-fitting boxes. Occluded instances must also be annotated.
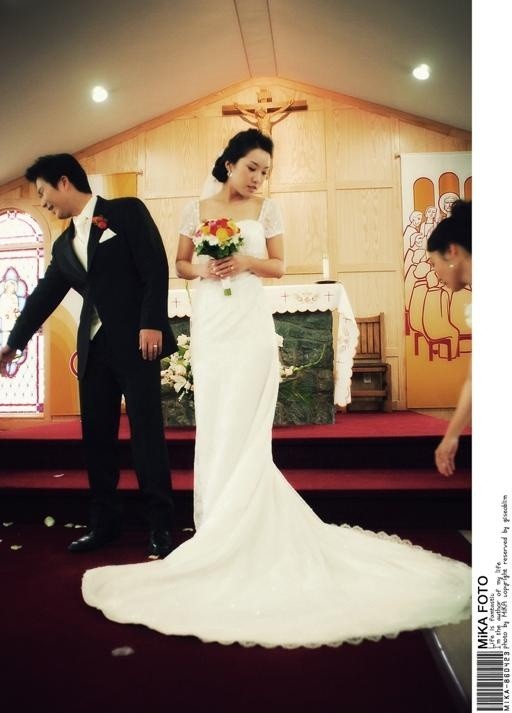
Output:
[68,524,121,553]
[149,529,173,556]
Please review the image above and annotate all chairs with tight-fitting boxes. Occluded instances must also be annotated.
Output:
[341,313,393,415]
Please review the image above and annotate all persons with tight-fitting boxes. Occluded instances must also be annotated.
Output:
[0,153,179,558]
[233,97,294,180]
[81,129,472,651]
[425,199,472,478]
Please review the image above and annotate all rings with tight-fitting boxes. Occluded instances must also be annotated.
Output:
[153,344,157,348]
[229,265,233,272]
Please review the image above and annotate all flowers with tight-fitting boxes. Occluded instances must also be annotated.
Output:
[191,217,245,296]
[92,214,109,230]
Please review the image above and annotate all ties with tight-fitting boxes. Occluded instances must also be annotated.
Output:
[74,216,102,341]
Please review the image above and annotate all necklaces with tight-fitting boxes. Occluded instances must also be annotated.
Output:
[221,202,242,220]
